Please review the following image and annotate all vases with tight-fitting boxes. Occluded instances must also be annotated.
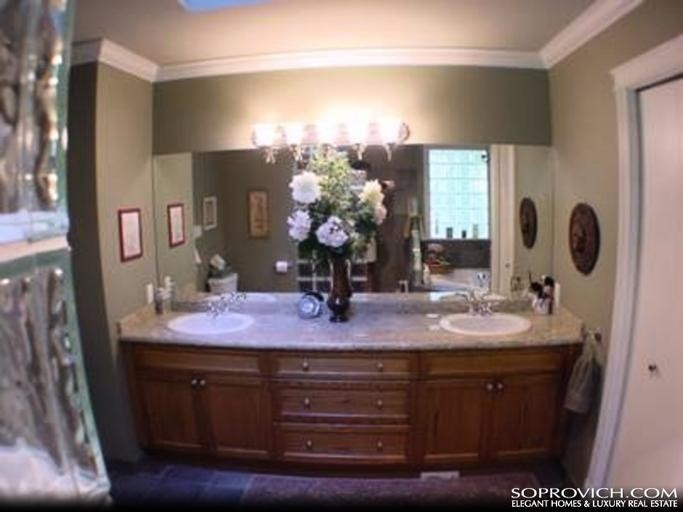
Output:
[325,246,352,322]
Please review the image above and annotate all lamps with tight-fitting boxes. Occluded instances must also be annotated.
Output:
[253,120,310,164]
[342,116,410,162]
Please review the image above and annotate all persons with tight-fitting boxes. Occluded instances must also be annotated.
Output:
[355,162,392,291]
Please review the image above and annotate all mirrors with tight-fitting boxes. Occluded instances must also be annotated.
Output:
[150,144,555,311]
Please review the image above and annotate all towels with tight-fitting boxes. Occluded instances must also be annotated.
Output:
[565,324,606,415]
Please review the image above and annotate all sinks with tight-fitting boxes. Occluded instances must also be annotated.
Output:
[165,311,254,335]
[439,312,532,337]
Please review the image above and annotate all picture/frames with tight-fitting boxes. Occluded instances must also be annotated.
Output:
[118,209,142,261]
[202,196,218,231]
[247,190,270,238]
[167,204,184,246]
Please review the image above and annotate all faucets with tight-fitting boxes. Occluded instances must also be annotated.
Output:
[469,288,493,317]
[206,296,231,316]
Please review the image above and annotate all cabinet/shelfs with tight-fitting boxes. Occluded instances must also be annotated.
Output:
[120,342,274,471]
[270,349,421,475]
[417,344,582,474]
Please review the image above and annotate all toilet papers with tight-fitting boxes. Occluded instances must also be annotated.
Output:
[275,260,289,273]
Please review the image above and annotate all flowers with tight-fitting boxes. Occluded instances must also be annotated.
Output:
[287,140,387,251]
